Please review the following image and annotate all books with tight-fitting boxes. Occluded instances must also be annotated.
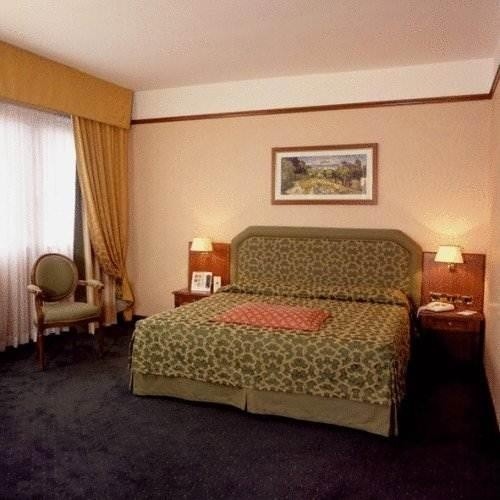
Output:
[190,270,213,293]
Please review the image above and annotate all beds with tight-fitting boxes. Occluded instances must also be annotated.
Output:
[124,223,427,444]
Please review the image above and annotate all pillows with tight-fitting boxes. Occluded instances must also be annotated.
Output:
[216,275,410,314]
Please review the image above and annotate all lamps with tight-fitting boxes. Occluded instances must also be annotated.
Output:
[433,244,464,270]
[189,233,218,261]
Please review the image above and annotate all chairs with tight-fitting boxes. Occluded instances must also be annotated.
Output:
[20,252,110,374]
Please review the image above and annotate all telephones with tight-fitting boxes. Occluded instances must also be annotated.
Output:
[425,301,455,312]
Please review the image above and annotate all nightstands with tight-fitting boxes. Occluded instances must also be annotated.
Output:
[172,241,232,314]
[418,252,489,360]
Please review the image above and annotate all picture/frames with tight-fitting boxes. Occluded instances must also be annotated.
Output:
[269,140,382,208]
